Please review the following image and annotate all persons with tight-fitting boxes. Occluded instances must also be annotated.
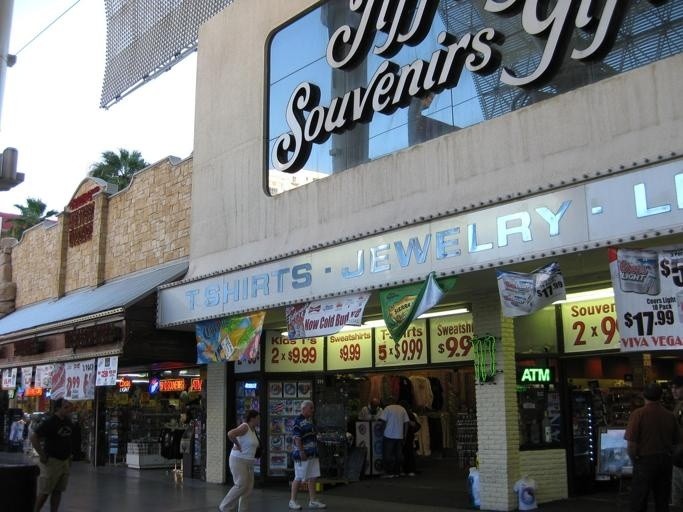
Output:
[669,377,682,512]
[30,398,70,512]
[218,409,260,512]
[397,398,421,477]
[357,397,383,422]
[286,399,327,511]
[380,395,409,476]
[621,381,679,510]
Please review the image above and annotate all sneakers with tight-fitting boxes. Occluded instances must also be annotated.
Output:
[289,500,302,509]
[308,500,326,508]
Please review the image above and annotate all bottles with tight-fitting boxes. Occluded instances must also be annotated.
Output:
[541,411,552,442]
[531,418,541,445]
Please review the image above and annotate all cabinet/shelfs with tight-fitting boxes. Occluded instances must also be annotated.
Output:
[607,379,653,428]
[105,390,184,468]
[235,377,348,487]
[455,411,477,477]
